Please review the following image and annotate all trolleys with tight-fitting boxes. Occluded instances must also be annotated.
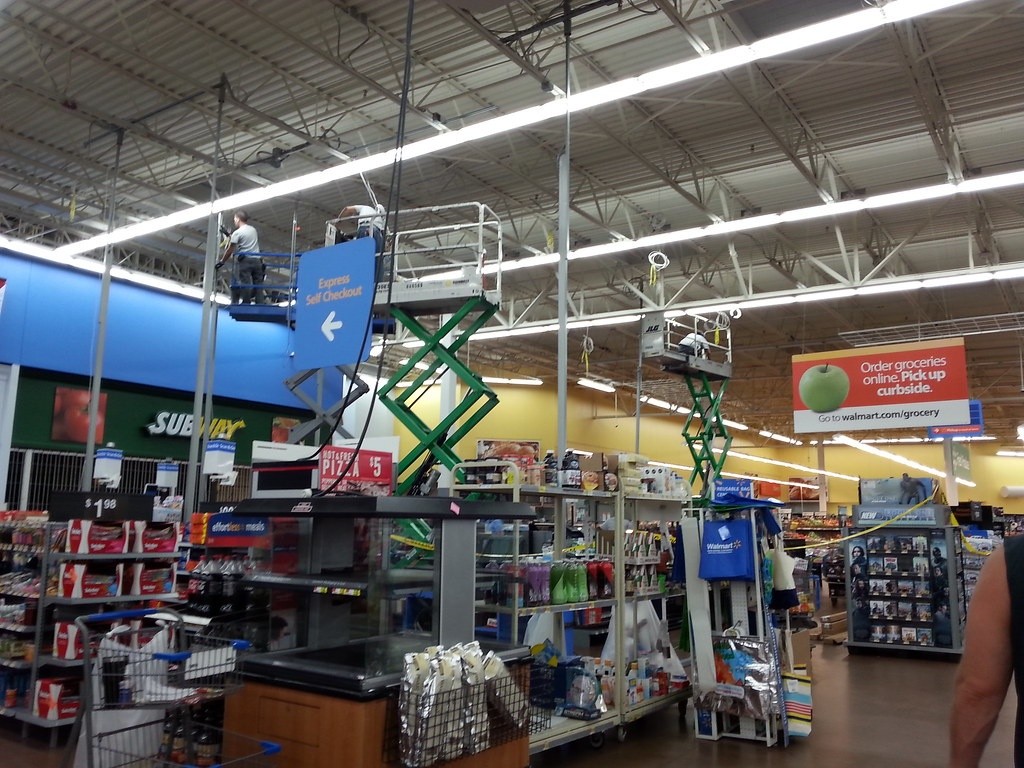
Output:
[77,604,284,768]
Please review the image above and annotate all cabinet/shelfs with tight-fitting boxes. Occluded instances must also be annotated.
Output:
[841,503,966,661]
[447,457,692,756]
[0,509,182,748]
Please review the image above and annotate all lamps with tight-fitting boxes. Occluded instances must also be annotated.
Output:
[577,376,616,393]
[996,450,1024,458]
[810,434,997,447]
[481,376,541,387]
[758,424,803,447]
[635,391,749,431]
[831,433,977,489]
[682,440,861,484]
[565,448,819,492]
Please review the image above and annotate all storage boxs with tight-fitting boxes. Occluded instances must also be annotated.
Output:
[32,678,80,720]
[126,563,177,595]
[65,519,128,555]
[58,563,123,599]
[53,623,121,661]
[123,520,180,552]
[582,471,619,492]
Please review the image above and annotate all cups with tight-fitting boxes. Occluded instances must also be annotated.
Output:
[102,656,129,710]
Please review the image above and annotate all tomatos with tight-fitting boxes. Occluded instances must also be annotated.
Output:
[54,387,107,443]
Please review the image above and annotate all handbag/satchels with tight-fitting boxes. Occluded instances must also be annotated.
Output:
[601,599,685,682]
[73,620,169,768]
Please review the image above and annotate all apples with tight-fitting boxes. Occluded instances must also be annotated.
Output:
[799,364,849,412]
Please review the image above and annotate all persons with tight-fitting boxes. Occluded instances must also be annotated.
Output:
[215,209,265,305]
[678,331,712,360]
[849,537,948,648]
[342,205,385,283]
[898,473,925,506]
[948,535,1024,768]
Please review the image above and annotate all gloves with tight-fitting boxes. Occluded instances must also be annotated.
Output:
[215,260,225,271]
[219,225,230,237]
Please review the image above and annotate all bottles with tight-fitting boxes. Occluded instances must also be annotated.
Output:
[192,625,262,654]
[585,657,670,706]
[483,557,615,607]
[188,556,272,605]
[543,451,581,489]
[160,699,222,767]
[119,676,135,708]
